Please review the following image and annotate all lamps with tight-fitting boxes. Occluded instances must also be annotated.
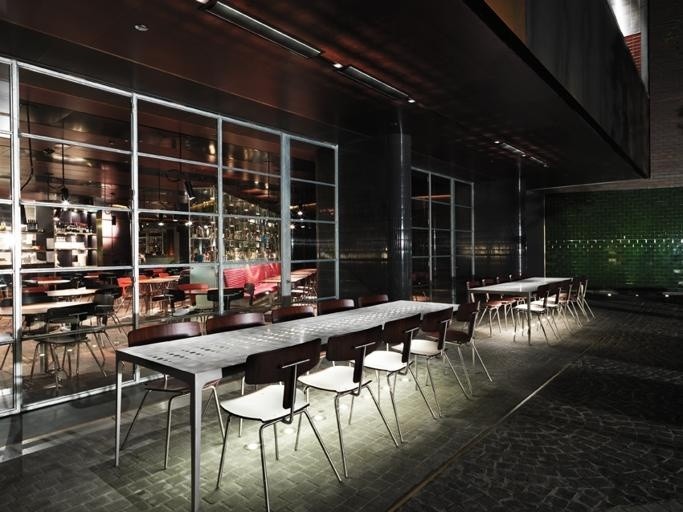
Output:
[157,213,165,226]
[61,142,71,210]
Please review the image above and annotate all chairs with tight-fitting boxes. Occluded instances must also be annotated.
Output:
[467,275,595,344]
[28,294,126,378]
[115,273,169,313]
[177,263,280,309]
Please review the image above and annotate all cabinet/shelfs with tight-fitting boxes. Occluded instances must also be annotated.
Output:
[190,187,279,263]
[139,232,164,256]
[0,203,97,269]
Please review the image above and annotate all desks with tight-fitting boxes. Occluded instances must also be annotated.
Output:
[0,299,89,374]
[260,269,316,308]
[48,286,95,298]
[139,276,179,316]
[117,301,460,511]
[24,280,70,283]
